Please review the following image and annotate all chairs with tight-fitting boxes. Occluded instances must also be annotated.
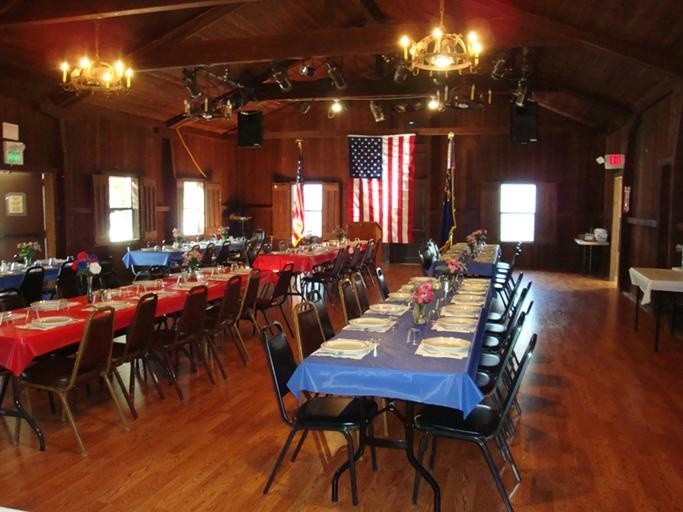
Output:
[0,226,538,512]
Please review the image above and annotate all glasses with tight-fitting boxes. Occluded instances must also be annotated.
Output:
[398,1,482,78]
[56,17,135,95]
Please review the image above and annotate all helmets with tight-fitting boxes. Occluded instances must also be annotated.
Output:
[445,278,488,314]
[32,316,72,327]
[28,266,253,323]
[439,316,477,330]
[368,276,434,313]
[347,317,388,329]
[421,337,470,351]
[321,339,370,353]
[439,244,497,261]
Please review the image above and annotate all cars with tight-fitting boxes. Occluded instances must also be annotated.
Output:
[11,261,20,271]
[426,311,439,327]
[406,327,421,346]
[228,236,233,243]
[154,245,159,252]
[66,255,74,262]
[370,337,382,358]
[48,257,57,266]
[286,237,361,255]
[34,260,42,268]
[389,317,401,335]
[0,261,8,272]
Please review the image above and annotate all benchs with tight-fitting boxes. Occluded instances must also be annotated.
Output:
[346,136,414,244]
[291,140,305,245]
[440,133,458,247]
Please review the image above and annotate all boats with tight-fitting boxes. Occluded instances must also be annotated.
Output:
[238,110,263,148]
[510,99,538,144]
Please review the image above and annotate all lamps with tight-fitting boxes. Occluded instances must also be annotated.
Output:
[56,17,135,95]
[180,51,530,120]
[398,1,482,78]
